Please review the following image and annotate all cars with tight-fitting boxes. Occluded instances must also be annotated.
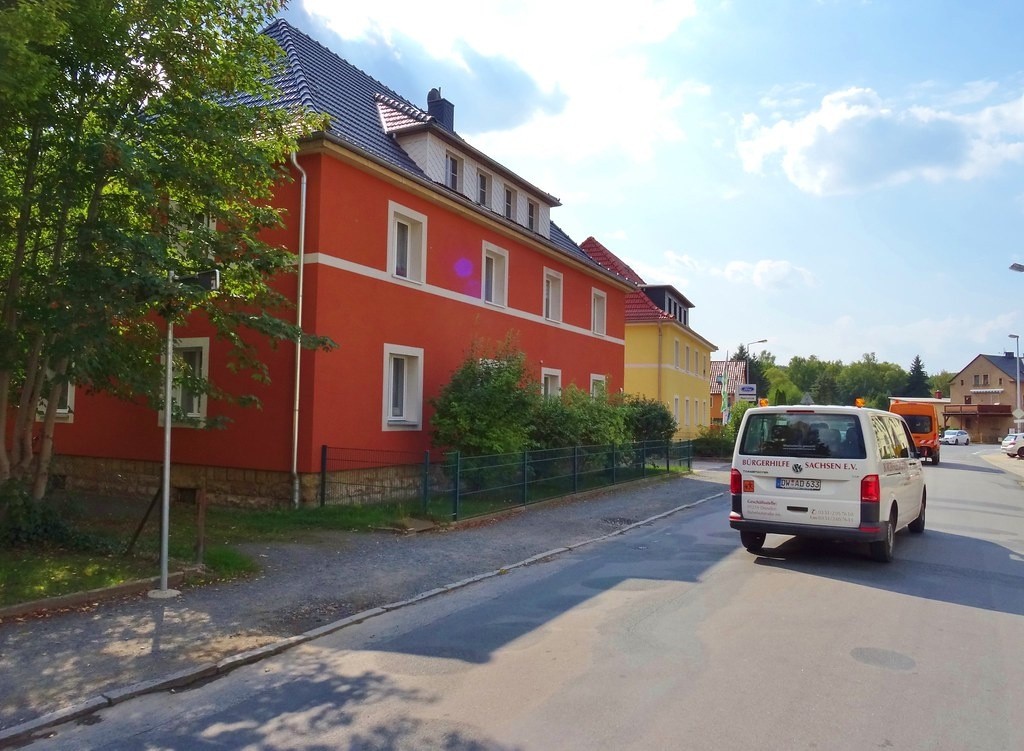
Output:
[939,430,971,446]
[1001,433,1024,458]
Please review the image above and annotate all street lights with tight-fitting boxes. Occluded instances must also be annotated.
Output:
[1008,335,1021,433]
[746,340,768,383]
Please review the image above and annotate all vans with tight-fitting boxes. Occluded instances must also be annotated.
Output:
[728,404,932,561]
[888,401,945,464]
